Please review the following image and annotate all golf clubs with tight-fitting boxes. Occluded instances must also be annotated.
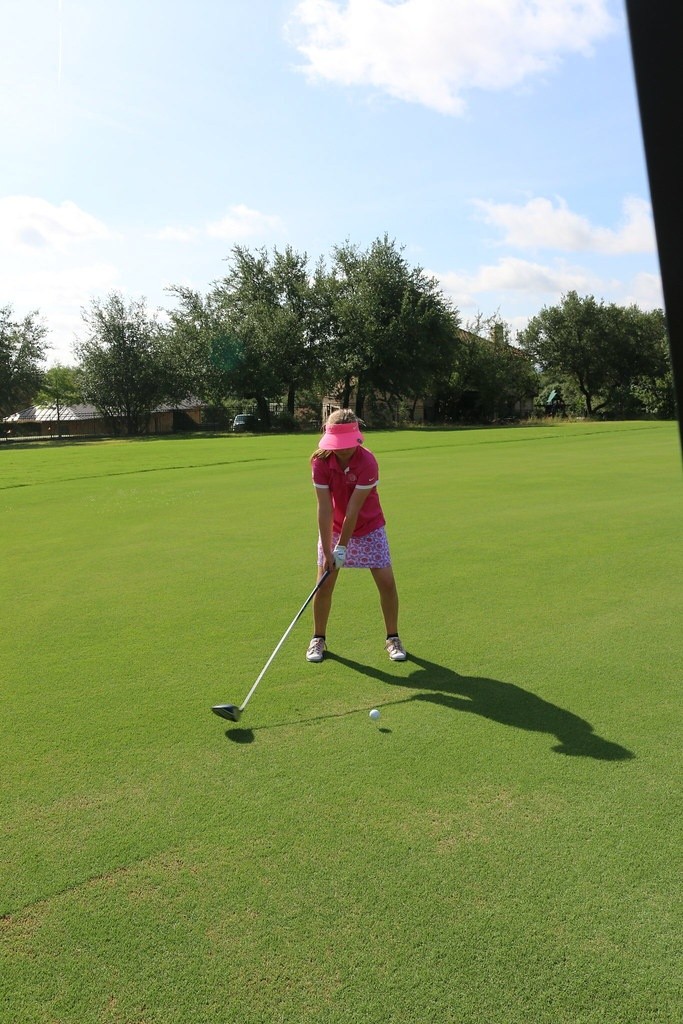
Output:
[212,559,336,723]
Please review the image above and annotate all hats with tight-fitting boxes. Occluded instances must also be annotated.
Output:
[318,421,364,450]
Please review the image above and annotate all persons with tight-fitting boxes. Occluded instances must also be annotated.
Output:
[304,409,407,664]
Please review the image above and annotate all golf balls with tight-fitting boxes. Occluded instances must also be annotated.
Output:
[369,709,380,720]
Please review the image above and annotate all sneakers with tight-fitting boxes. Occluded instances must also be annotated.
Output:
[306,638,327,662]
[384,637,406,661]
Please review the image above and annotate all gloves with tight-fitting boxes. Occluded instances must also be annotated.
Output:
[333,544,347,570]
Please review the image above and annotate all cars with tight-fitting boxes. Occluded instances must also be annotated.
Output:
[230,414,260,431]
